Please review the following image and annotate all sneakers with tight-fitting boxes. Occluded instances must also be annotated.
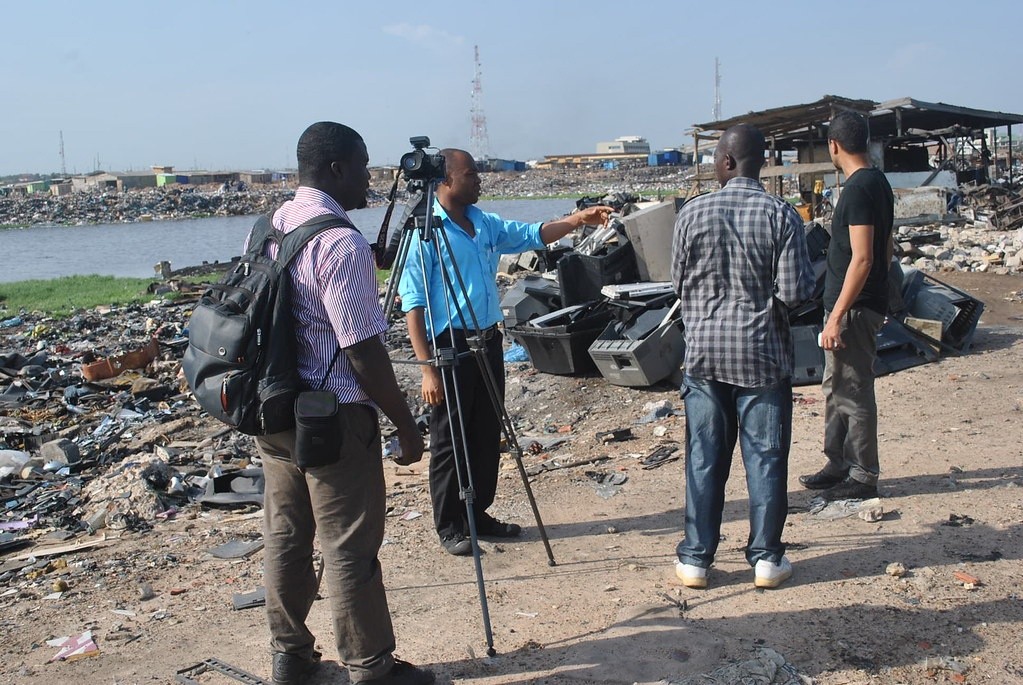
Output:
[753,555,793,588]
[439,532,473,556]
[820,473,879,501]
[477,511,521,538]
[673,558,712,588]
[797,469,834,489]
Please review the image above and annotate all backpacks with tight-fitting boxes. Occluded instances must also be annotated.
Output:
[183,209,363,437]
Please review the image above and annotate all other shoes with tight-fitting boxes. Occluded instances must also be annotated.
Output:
[271,650,323,685]
[348,657,436,685]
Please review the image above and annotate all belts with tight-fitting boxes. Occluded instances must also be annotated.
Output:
[854,297,882,308]
[438,325,499,341]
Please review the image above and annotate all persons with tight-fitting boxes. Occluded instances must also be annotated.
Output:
[243,121,434,684]
[799,113,894,499]
[398,148,615,553]
[670,124,803,587]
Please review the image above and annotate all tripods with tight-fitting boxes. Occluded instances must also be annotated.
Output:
[378,176,556,657]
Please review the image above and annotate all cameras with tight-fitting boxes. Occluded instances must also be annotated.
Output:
[400,136,447,183]
